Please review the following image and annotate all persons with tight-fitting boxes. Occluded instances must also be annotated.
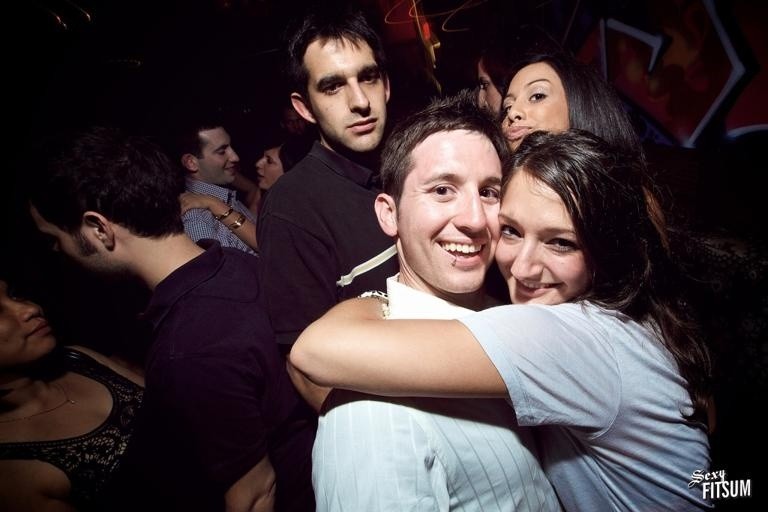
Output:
[0,4,768,512]
